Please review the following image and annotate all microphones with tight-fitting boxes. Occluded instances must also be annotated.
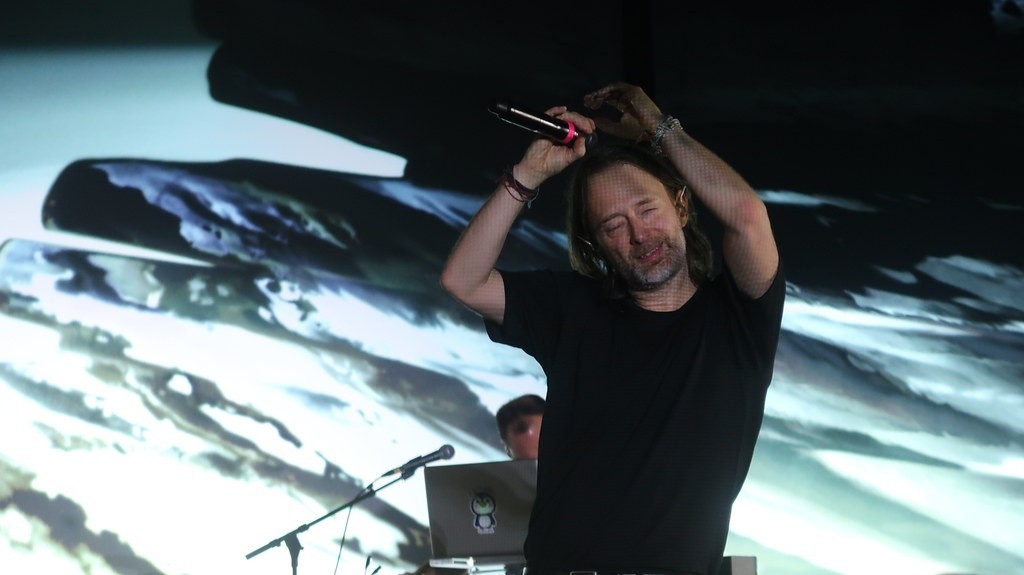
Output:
[383,445,455,478]
[487,101,596,149]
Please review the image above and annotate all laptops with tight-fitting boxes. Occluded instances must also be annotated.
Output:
[424,457,540,556]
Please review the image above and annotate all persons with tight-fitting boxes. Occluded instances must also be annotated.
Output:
[440,82,785,575]
[496,394,547,575]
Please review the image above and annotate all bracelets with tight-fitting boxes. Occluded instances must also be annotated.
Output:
[653,117,681,155]
[502,167,539,205]
